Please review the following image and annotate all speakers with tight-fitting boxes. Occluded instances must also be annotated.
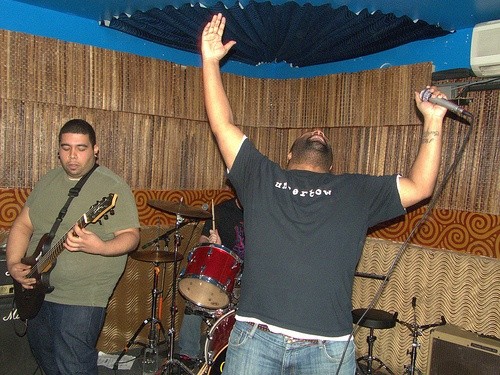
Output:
[426,325,500,375]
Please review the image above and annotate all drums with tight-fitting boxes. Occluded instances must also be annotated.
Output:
[177,241,244,311]
[204,308,239,375]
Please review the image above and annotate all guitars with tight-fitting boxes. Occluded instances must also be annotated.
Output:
[12,192,118,322]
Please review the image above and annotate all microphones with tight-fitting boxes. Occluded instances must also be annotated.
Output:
[419,89,474,123]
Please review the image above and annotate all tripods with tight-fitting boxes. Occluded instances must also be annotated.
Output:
[157,214,194,375]
[111,263,173,371]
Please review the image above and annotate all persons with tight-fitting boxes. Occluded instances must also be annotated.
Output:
[201,12,447,375]
[6,119,141,375]
[171,196,246,375]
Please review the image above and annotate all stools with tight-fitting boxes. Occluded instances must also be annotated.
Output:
[352,308,396,375]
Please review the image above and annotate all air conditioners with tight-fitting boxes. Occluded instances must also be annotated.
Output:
[470,19,500,78]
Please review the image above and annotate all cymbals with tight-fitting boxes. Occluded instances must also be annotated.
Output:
[128,250,184,263]
[146,199,213,220]
